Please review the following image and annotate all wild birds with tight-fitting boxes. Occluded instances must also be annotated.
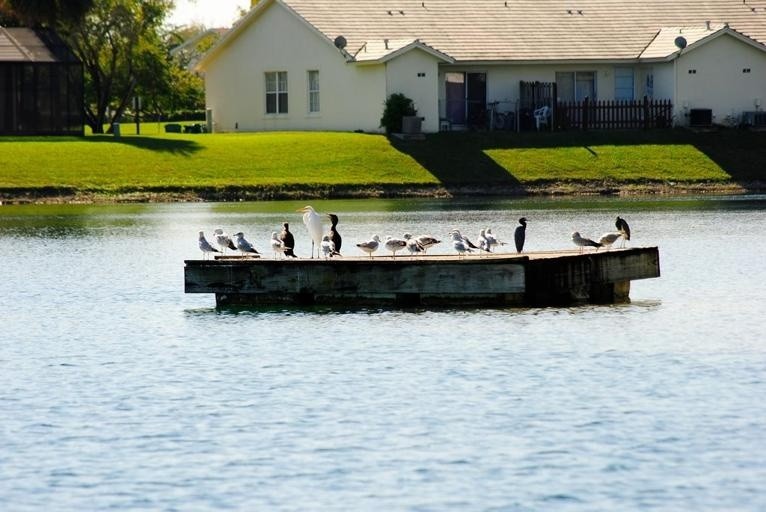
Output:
[596,232,622,252]
[571,231,605,255]
[448,228,508,255]
[199,231,223,260]
[615,216,630,248]
[356,235,381,259]
[383,232,441,260]
[233,232,260,260]
[297,206,342,260]
[271,222,298,259]
[213,229,238,254]
[514,217,532,254]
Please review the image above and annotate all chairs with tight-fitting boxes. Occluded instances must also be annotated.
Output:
[534,106,549,132]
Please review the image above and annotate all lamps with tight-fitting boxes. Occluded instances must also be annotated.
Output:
[675,37,687,57]
[335,36,347,58]
